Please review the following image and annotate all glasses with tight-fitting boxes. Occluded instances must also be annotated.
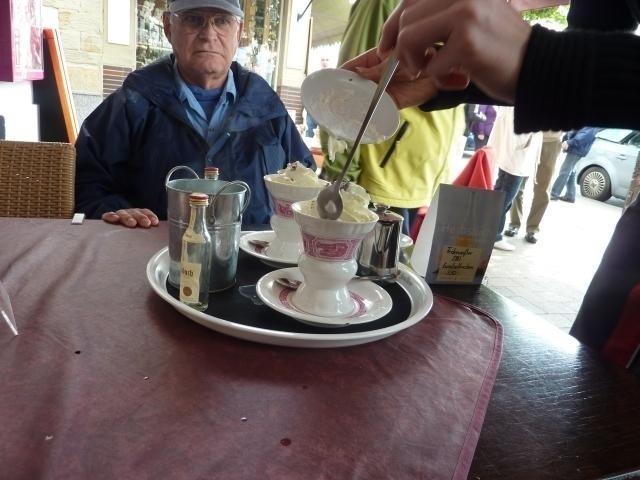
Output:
[167,9,242,36]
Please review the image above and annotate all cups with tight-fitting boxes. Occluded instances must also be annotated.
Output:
[290,198,380,318]
[261,171,330,261]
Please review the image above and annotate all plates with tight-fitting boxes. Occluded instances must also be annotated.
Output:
[300,68,400,145]
[254,267,394,328]
[241,228,300,268]
[145,230,436,349]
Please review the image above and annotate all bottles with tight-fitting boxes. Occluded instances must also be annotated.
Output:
[179,192,210,313]
[204,167,218,182]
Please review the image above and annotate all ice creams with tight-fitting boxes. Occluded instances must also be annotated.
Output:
[264,160,332,189]
[290,195,379,224]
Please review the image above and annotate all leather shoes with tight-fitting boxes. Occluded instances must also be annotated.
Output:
[557,196,576,203]
[549,194,559,200]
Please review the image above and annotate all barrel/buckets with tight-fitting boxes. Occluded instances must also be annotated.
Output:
[165,165,250,292]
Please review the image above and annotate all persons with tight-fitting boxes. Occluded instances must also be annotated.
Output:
[472,103,498,164]
[343,0,640,366]
[489,106,543,248]
[504,130,560,245]
[319,0,568,263]
[549,125,598,202]
[71,0,317,229]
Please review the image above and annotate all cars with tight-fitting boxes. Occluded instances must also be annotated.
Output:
[575,128,640,202]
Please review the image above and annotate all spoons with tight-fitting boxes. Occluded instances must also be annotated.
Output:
[315,58,401,221]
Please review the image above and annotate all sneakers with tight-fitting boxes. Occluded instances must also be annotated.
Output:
[504,226,519,236]
[492,241,516,251]
[524,232,538,244]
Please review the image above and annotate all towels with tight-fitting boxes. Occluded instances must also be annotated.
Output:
[410,146,493,246]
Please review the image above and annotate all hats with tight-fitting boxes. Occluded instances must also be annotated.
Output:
[165,0,246,19]
